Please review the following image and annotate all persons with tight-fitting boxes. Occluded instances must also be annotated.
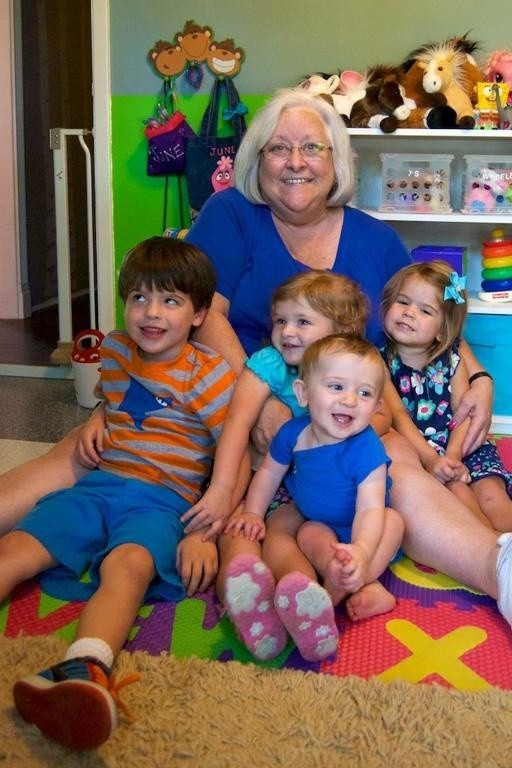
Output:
[378,259,512,534]
[179,268,392,663]
[0,89,512,627]
[0,235,253,751]
[223,331,404,622]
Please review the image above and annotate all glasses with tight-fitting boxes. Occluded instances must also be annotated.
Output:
[258,138,333,162]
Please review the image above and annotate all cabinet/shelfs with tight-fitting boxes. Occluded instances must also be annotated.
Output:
[337,122,511,423]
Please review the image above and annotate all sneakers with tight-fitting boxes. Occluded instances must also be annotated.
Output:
[11,653,121,754]
[492,528,512,632]
[219,550,291,664]
[271,565,342,665]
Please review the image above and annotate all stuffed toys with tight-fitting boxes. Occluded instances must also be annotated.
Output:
[295,27,512,131]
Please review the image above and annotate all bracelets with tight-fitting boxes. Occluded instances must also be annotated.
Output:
[469,370,493,385]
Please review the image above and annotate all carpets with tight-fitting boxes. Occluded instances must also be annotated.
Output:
[0,428,512,768]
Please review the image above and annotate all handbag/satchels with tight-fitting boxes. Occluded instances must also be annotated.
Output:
[145,112,199,179]
[182,70,258,214]
